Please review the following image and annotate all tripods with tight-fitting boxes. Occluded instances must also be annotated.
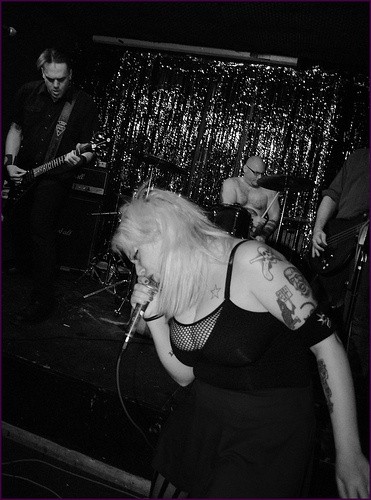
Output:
[70,174,135,317]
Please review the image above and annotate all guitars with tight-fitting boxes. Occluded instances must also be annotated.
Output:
[8,132,118,201]
[310,211,370,275]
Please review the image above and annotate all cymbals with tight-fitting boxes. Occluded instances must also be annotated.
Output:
[256,175,313,193]
[137,152,185,174]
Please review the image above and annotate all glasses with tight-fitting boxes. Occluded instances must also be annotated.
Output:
[247,166,267,177]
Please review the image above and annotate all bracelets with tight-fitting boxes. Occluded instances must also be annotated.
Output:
[144,312,166,322]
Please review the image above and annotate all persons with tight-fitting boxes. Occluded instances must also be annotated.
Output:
[220,156,280,243]
[311,149,371,372]
[0,51,98,325]
[110,188,370,500]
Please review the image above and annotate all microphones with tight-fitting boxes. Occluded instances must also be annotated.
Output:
[122,275,160,349]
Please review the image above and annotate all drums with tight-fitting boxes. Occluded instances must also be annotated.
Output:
[209,203,252,239]
[96,246,130,273]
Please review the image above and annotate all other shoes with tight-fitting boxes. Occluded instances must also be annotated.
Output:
[9,293,55,326]
[6,266,30,278]
